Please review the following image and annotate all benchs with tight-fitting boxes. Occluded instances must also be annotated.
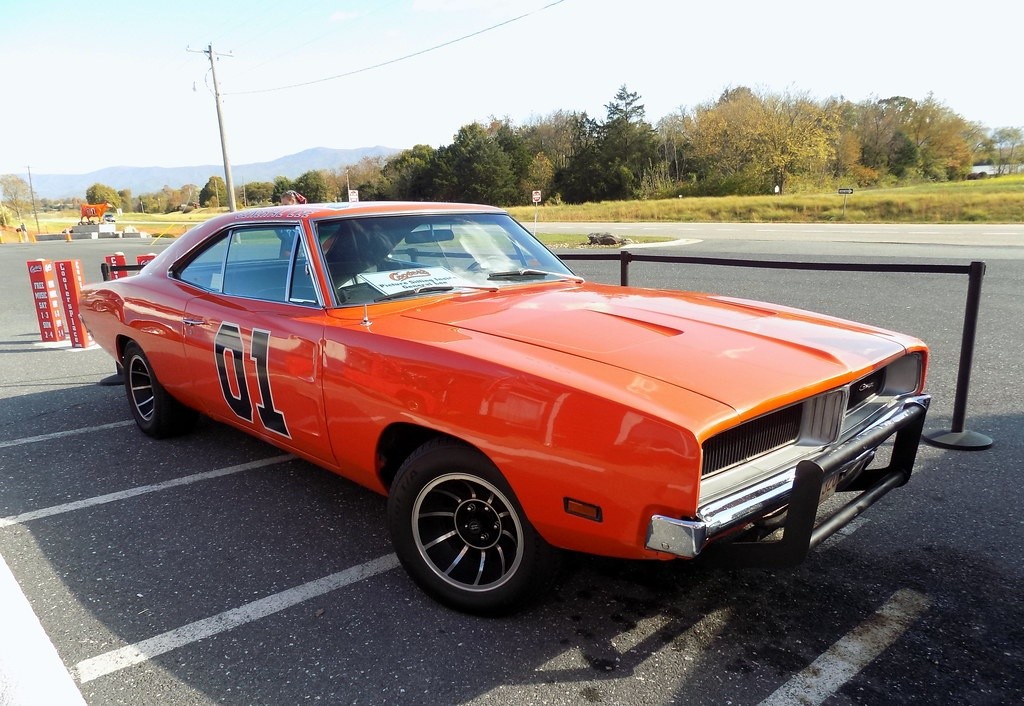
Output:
[190,264,307,296]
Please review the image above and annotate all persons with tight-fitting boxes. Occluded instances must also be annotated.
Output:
[273,190,309,259]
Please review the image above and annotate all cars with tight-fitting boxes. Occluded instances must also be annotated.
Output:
[103,214,116,223]
[77,199,933,617]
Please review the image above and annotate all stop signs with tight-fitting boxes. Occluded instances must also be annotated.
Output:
[531,191,544,203]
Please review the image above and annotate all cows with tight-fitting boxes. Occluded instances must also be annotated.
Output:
[80,201,113,223]
